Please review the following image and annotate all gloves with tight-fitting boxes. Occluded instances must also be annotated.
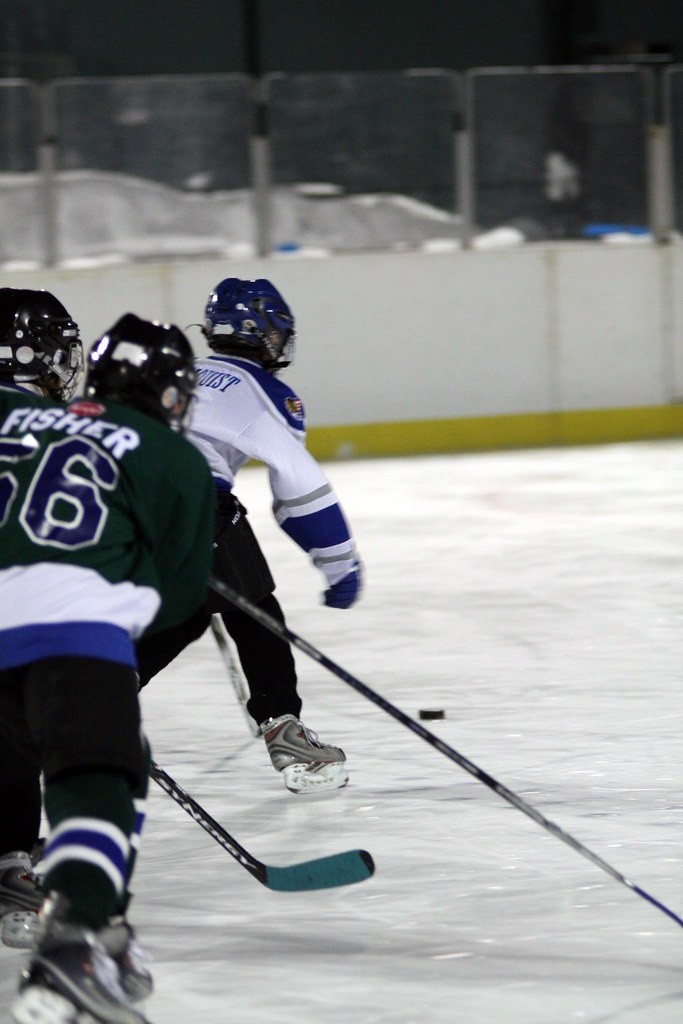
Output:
[324,574,358,610]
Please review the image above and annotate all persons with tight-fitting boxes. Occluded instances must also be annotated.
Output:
[0,287,212,1024]
[137,277,362,794]
[544,149,590,238]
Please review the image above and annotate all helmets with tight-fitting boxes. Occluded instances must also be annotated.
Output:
[84,313,197,431]
[206,275,296,368]
[0,287,83,406]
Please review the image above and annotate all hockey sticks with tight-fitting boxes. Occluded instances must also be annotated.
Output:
[145,759,377,895]
[210,614,263,739]
[209,578,683,929]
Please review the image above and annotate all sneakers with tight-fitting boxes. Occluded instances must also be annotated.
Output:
[261,712,349,793]
[9,927,151,1024]
[102,915,152,1001]
[0,851,46,950]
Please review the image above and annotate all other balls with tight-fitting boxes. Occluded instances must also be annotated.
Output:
[418,708,445,721]
